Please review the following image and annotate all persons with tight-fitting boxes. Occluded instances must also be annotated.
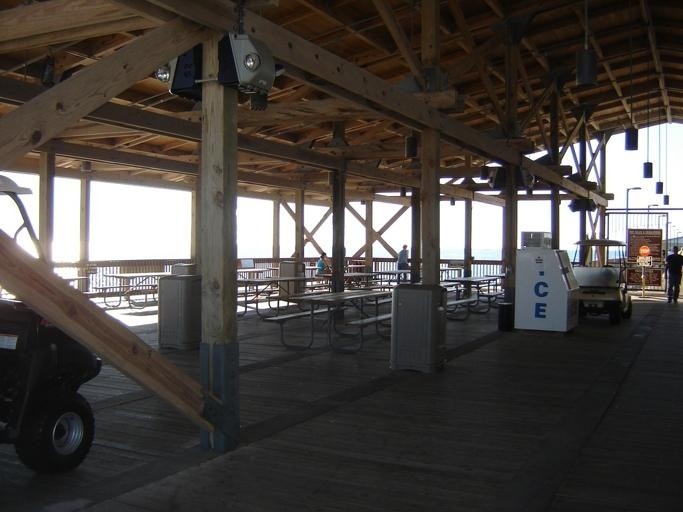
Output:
[315,252,331,274]
[398,245,410,270]
[664,246,683,305]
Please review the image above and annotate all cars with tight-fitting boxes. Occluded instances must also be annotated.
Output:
[0,175,104,475]
[570,240,633,327]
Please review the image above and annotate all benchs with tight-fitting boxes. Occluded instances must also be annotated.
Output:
[439,257,503,319]
[92,259,171,310]
[234,260,422,356]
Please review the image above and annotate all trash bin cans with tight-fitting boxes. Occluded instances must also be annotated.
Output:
[499,303,514,332]
[279,261,305,296]
[159,274,201,350]
[391,284,446,374]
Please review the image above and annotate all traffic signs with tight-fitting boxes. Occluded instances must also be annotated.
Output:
[636,256,652,267]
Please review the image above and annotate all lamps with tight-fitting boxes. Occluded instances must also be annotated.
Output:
[578,3,670,205]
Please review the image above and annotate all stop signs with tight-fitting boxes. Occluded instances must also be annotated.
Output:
[638,246,649,256]
